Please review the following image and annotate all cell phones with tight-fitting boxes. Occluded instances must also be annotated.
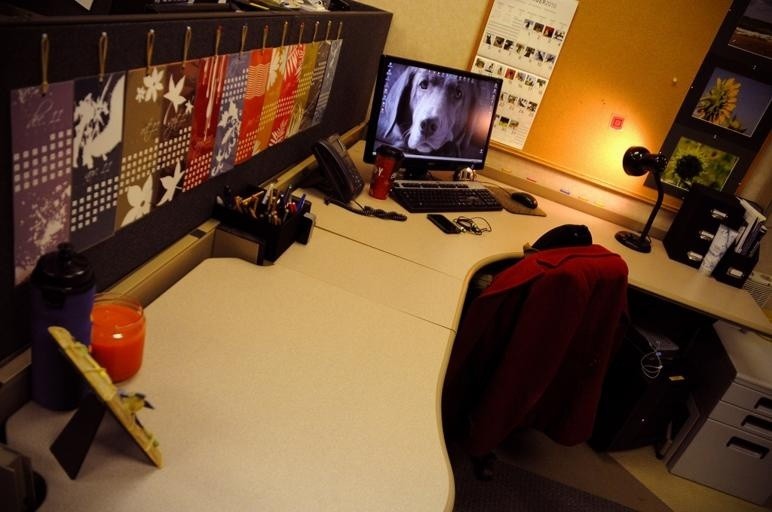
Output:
[427,214,461,234]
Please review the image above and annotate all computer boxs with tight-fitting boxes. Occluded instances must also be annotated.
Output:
[585,320,709,453]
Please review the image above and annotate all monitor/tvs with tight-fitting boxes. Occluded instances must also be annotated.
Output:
[363,54,503,181]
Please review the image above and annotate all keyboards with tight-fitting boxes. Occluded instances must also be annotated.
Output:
[392,180,503,213]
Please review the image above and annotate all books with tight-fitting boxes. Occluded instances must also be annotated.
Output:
[731,194,768,261]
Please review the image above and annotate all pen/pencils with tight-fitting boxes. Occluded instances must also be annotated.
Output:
[216,179,307,225]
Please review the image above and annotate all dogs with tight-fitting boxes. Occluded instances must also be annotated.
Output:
[383,66,480,158]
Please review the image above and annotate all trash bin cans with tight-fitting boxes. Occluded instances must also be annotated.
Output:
[310,132,365,204]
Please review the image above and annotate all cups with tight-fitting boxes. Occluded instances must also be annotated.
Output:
[368,144,402,200]
[93,290,146,384]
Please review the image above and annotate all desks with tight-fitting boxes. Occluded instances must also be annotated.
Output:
[0,138,772,512]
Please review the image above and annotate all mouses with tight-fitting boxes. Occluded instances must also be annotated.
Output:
[511,192,537,209]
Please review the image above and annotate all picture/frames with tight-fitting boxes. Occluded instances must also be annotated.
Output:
[643,0,772,202]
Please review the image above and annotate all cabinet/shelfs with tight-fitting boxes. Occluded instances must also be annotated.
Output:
[0,0,393,369]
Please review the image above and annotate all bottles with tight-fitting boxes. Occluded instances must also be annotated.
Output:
[27,240,92,413]
